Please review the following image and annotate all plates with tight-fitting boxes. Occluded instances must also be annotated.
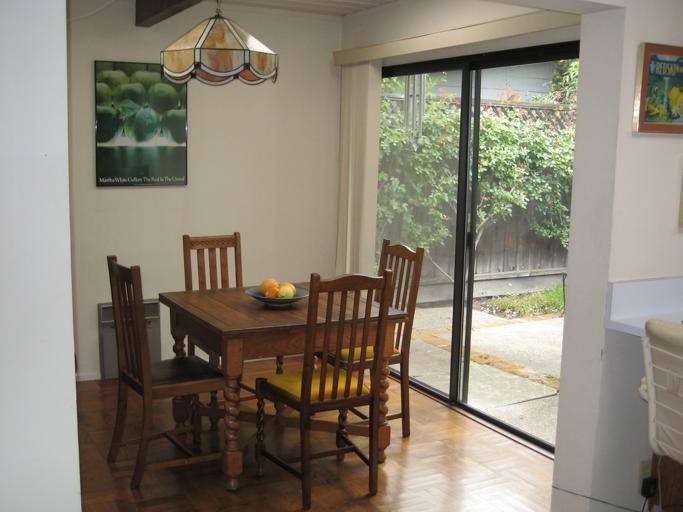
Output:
[244,284,310,309]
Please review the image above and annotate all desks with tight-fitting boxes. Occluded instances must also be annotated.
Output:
[158,283,411,495]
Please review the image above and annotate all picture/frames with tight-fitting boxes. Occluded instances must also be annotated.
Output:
[631,42,683,136]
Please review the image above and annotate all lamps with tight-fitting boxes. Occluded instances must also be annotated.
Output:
[160,1,279,86]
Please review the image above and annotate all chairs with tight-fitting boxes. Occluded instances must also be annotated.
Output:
[309,239,425,445]
[106,254,227,488]
[181,233,285,427]
[254,267,394,509]
[642,316,683,467]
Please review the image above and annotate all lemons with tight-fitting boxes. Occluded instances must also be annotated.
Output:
[276,285,294,299]
